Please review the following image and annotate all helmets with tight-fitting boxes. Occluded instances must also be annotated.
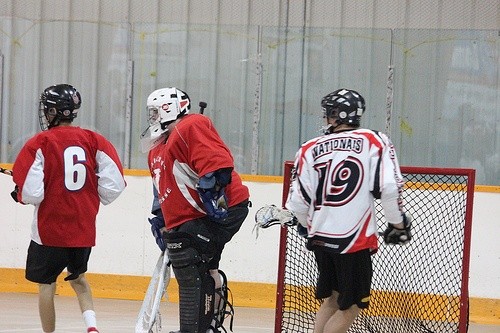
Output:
[321,89,365,135]
[38,84,81,131]
[147,87,191,140]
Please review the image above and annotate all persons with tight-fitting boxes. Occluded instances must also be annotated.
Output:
[140,87,252,333]
[285,89,412,333]
[11,84,127,333]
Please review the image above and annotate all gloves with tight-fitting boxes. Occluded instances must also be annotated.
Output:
[148,214,169,252]
[196,184,231,218]
[384,213,412,245]
[297,223,308,239]
[11,185,18,202]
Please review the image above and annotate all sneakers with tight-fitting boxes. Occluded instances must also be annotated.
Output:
[88,327,99,333]
